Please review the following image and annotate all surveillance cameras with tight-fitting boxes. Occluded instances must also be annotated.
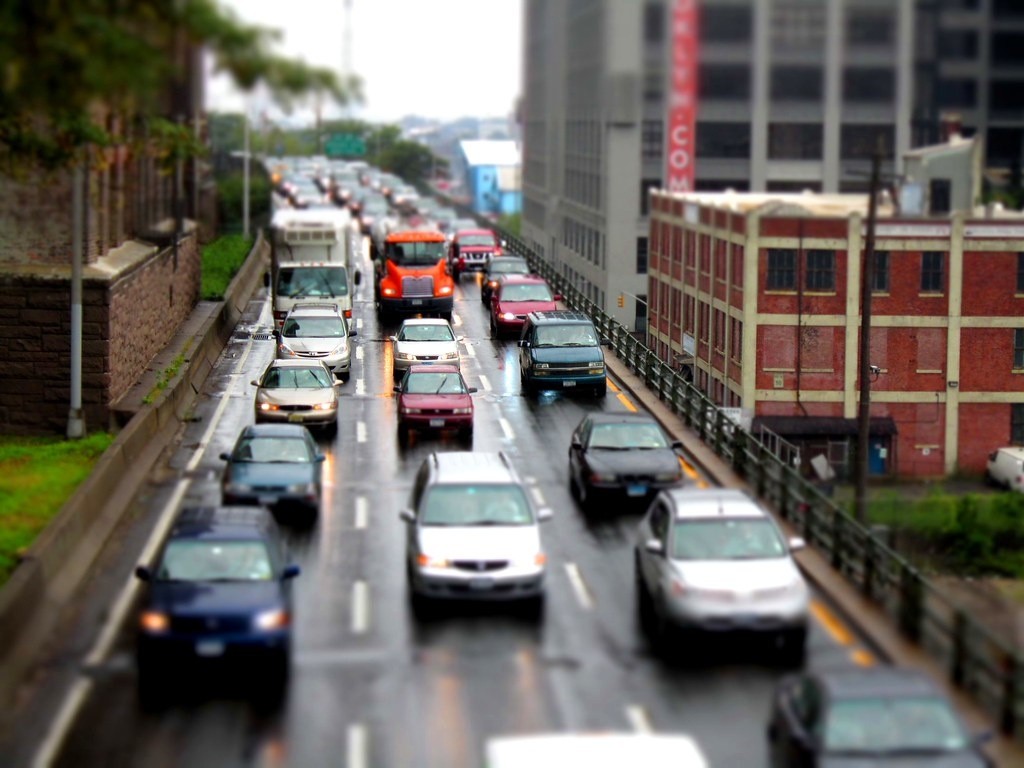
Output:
[870,366,878,371]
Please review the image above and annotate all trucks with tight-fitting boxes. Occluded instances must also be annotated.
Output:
[371,217,458,322]
[263,207,361,334]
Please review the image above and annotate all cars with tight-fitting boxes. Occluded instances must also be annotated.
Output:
[567,411,683,514]
[230,147,481,260]
[251,359,344,439]
[764,663,996,768]
[389,318,465,377]
[986,446,1024,496]
[394,365,479,444]
[218,423,326,524]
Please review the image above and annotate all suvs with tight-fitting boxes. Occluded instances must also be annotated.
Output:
[489,274,563,339]
[133,506,302,691]
[400,450,555,623]
[517,309,608,396]
[453,228,508,285]
[479,254,532,308]
[631,486,811,667]
[272,303,358,382]
